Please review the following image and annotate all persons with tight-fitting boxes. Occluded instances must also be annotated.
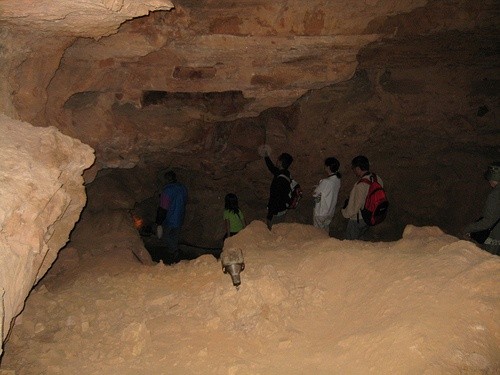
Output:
[264,146,294,231]
[339,155,384,242]
[460,165,500,257]
[222,192,247,242]
[311,157,342,234]
[155,170,188,261]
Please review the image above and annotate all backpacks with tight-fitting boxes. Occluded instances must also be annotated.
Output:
[278,173,303,210]
[356,172,388,226]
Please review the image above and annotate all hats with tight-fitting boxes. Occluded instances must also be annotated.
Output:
[483,163,500,182]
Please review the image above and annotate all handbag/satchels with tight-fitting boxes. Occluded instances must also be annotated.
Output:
[466,215,492,245]
